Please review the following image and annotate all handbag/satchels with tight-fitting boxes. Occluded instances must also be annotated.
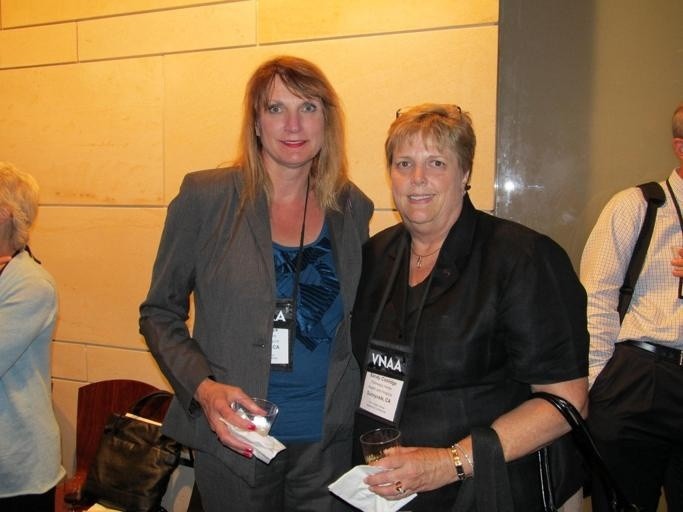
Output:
[80,411,199,512]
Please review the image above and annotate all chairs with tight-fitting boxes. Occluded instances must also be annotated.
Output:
[51,376,174,512]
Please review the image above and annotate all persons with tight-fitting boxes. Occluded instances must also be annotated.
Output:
[579,103,683,511]
[350,103,589,512]
[139,55,374,512]
[0,161,66,512]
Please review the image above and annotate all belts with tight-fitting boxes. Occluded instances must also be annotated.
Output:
[624,339,682,365]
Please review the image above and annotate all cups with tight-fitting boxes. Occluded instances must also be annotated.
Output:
[670,242,682,271]
[230,397,278,434]
[358,428,400,468]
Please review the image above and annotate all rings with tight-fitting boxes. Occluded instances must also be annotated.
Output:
[396,486,404,494]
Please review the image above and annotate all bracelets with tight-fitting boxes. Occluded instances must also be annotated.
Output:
[451,446,465,480]
[454,443,473,469]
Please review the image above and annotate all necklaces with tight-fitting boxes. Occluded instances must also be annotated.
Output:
[411,247,440,268]
